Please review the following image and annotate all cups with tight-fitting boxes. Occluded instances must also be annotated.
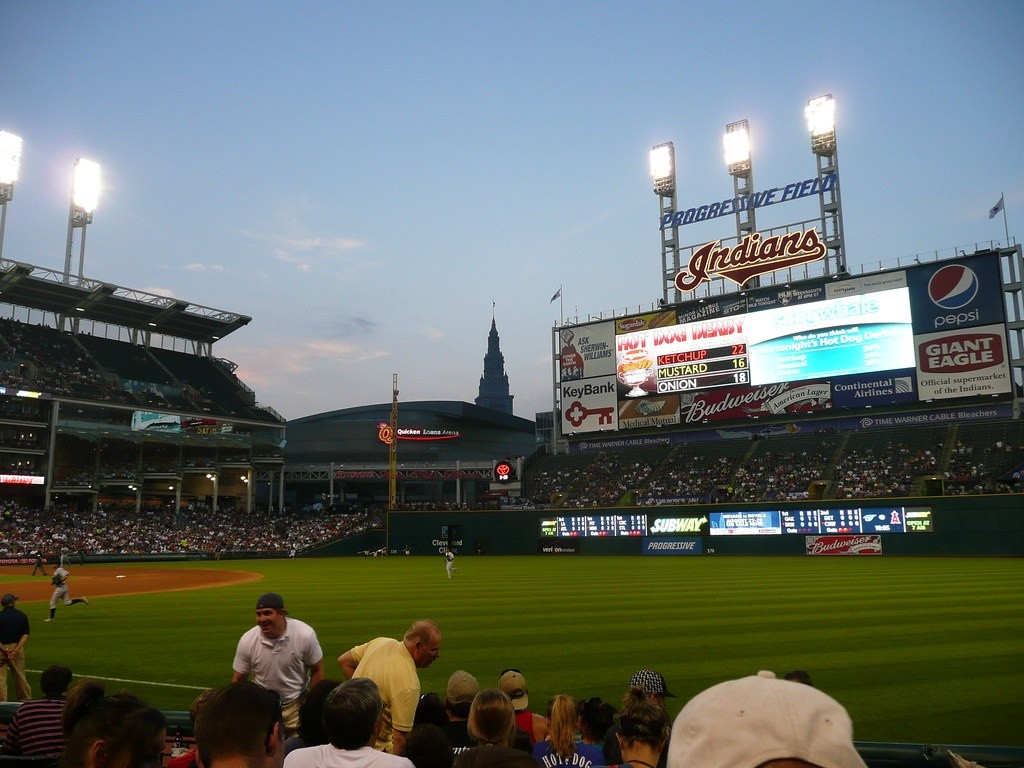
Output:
[172,748,184,757]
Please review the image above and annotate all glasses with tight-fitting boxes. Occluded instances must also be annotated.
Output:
[420,694,425,703]
[585,696,601,708]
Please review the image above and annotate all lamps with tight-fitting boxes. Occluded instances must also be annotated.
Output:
[960,249,968,258]
[569,431,580,437]
[657,424,664,429]
[914,258,922,265]
[599,429,607,433]
[703,418,712,425]
[565,321,575,325]
[620,313,627,318]
[592,316,601,321]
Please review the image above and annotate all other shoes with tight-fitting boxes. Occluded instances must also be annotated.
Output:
[44,618,55,623]
[80,597,89,606]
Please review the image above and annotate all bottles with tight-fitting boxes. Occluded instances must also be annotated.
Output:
[174,724,184,748]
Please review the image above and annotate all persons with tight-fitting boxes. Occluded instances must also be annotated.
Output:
[0,315,1024,578]
[233,592,323,739]
[0,619,988,768]
[0,594,32,702]
[44,560,90,622]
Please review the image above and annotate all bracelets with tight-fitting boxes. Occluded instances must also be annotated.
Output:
[4,649,8,652]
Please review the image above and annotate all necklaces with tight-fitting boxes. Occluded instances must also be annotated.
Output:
[625,760,654,768]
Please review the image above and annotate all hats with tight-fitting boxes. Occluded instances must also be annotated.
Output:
[668,670,867,768]
[256,593,289,616]
[447,670,479,705]
[630,669,677,697]
[52,559,61,564]
[499,669,529,710]
[40,665,72,693]
[1,593,19,606]
